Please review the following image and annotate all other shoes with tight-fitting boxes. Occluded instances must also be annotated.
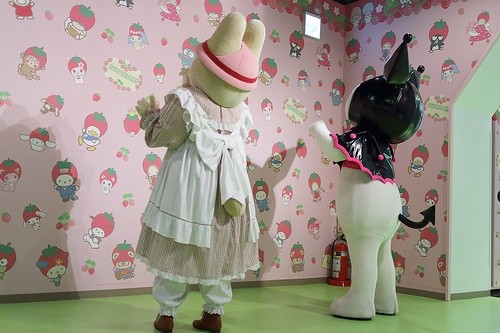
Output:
[193,310,222,332]
[153,315,174,333]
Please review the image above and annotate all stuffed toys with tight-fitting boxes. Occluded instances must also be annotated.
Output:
[309,34,426,321]
[134,11,266,333]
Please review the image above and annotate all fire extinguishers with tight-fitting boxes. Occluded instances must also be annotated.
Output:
[327,234,351,286]
